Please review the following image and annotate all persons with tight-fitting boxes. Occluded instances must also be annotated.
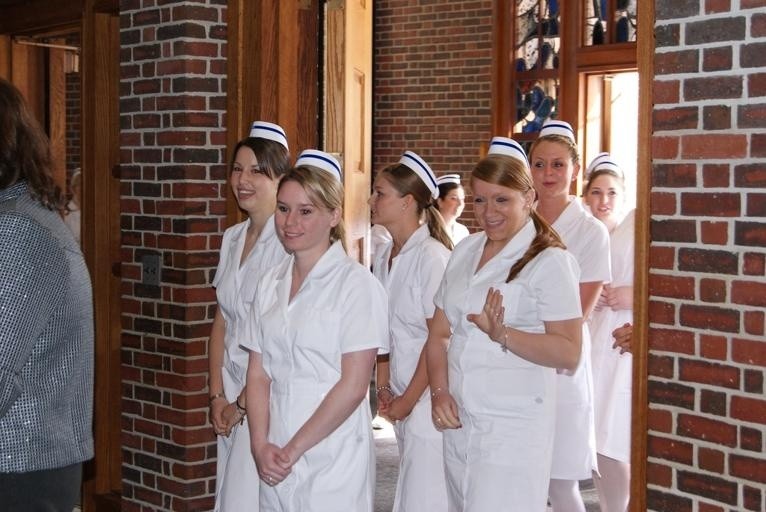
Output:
[63,165,81,246]
[203,119,637,512]
[0,77,97,512]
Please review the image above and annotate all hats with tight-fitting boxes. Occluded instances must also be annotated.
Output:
[435,172,461,186]
[400,147,441,201]
[581,152,627,181]
[487,135,531,178]
[247,118,290,151]
[537,120,578,145]
[292,147,343,185]
